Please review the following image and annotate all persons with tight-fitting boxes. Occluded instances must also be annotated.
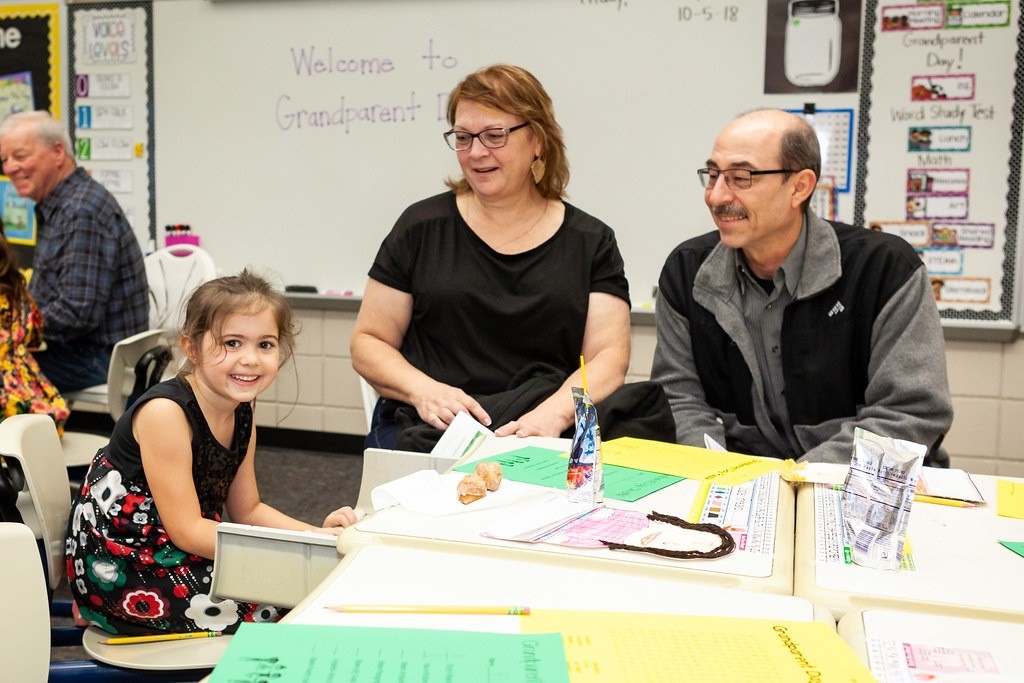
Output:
[63,267,361,637]
[349,61,634,453]
[0,218,70,454]
[650,105,955,472]
[0,107,149,391]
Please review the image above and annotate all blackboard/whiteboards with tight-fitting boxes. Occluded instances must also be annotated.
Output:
[66,0,1023,340]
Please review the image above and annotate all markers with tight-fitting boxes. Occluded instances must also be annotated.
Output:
[320,289,353,297]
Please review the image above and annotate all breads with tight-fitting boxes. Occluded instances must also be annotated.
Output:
[457,461,502,505]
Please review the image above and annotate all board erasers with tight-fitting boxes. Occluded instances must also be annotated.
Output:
[285,285,318,293]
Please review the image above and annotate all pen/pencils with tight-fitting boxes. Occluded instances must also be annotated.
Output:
[323,604,532,616]
[825,484,977,508]
[97,631,222,645]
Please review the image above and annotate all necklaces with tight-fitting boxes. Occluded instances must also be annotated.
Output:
[465,191,549,249]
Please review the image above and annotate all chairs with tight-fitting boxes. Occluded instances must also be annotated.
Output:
[53,243,218,413]
[0,414,236,683]
[15,329,173,481]
[0,523,51,683]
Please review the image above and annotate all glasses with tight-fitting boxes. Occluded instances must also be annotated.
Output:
[443,122,530,151]
[697,168,798,190]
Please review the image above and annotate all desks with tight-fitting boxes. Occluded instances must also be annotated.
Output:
[335,434,796,598]
[837,609,1024,683]
[797,461,1024,623]
[201,546,836,683]
